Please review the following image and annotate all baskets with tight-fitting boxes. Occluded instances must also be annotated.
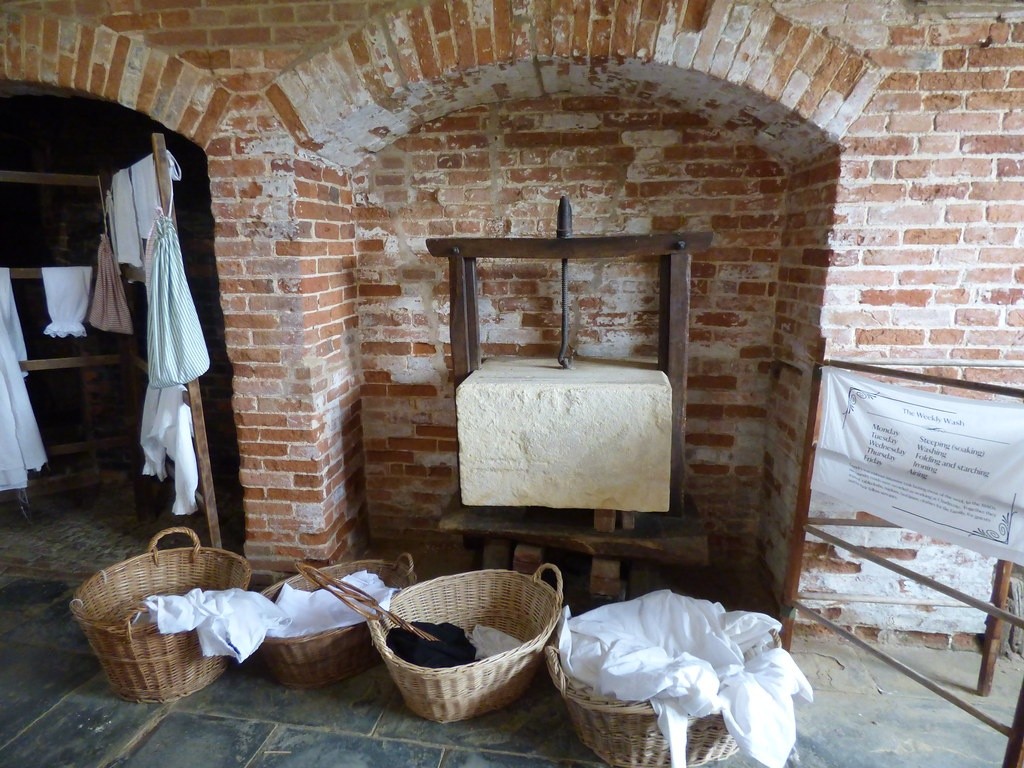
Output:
[72,525,254,702]
[368,558,569,721]
[550,599,784,767]
[254,550,417,688]
